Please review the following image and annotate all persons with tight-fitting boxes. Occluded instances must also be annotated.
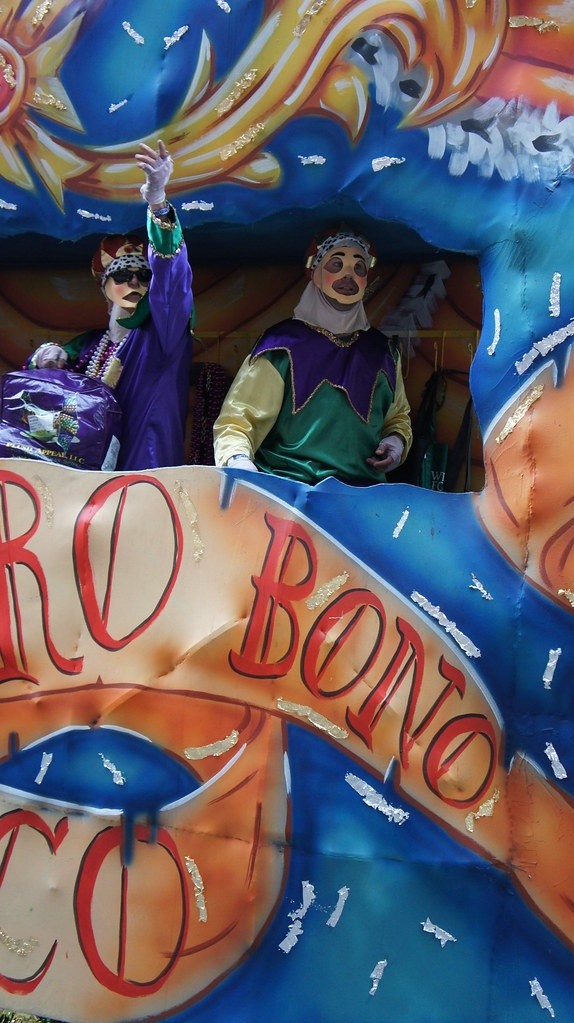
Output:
[24,139,193,470]
[212,232,412,484]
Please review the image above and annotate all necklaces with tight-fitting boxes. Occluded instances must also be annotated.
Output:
[69,330,126,378]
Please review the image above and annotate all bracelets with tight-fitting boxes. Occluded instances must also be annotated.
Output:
[153,204,169,217]
